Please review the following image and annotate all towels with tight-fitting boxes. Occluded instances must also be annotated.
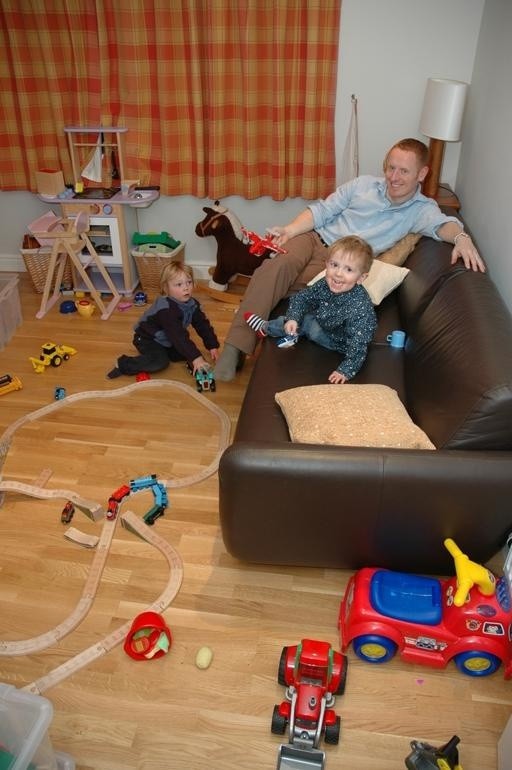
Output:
[339,100,358,185]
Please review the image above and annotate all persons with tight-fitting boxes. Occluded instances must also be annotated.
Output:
[243,235,378,384]
[213,138,487,382]
[106,261,220,380]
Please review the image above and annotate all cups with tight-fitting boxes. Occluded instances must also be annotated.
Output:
[386,330,406,348]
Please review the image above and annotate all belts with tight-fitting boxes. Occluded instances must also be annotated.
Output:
[312,229,329,248]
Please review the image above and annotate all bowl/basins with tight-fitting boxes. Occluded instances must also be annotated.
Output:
[62,300,76,314]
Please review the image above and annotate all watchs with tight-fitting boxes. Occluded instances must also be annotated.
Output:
[453,232,472,245]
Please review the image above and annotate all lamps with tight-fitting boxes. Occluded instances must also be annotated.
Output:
[415,76,470,208]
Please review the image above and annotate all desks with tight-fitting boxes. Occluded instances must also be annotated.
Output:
[424,183,463,217]
[29,183,162,301]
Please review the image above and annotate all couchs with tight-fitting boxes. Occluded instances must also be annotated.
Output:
[214,198,512,578]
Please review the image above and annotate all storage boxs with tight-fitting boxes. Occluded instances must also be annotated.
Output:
[54,750,78,770]
[0,272,25,349]
[1,680,57,769]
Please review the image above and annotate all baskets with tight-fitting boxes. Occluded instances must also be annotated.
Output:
[130,241,186,295]
[19,240,73,294]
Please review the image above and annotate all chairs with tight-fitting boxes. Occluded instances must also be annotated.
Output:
[28,209,126,324]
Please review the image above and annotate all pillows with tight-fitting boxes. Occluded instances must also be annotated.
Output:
[270,377,443,451]
[376,230,422,269]
[306,255,410,304]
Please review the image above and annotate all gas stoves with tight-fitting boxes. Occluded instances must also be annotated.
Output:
[64,185,122,218]
